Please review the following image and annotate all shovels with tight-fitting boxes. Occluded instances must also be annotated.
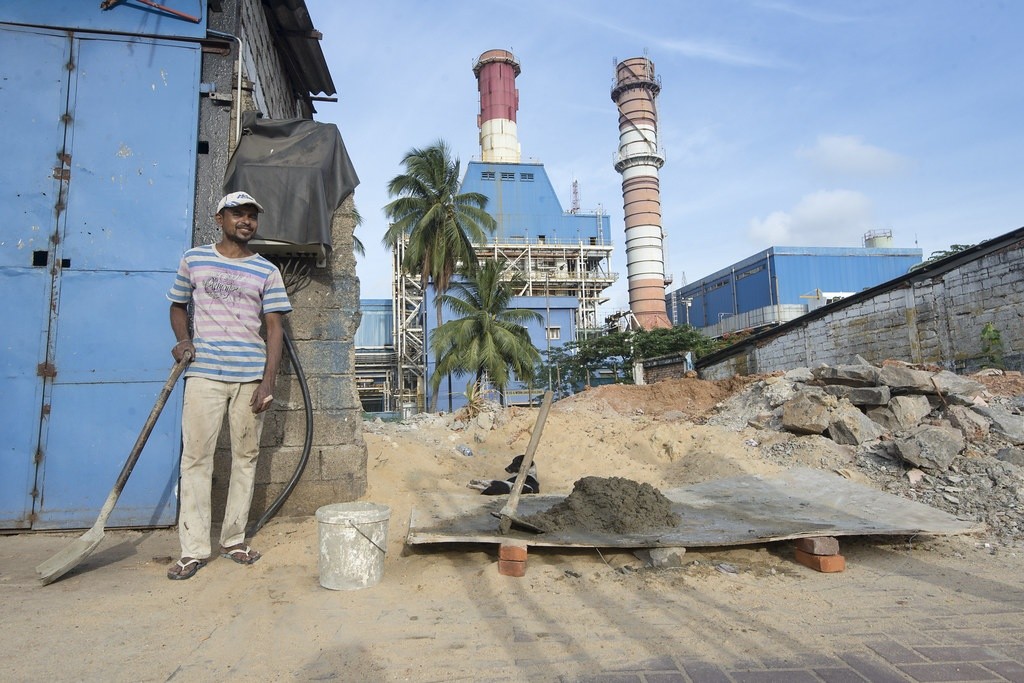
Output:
[34,351,194,585]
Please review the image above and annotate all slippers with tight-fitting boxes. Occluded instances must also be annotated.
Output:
[167,557,208,580]
[220,546,262,564]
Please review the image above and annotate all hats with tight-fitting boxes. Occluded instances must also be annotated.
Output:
[217,191,264,214]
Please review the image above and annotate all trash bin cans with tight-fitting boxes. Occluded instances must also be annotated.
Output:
[402,401,417,421]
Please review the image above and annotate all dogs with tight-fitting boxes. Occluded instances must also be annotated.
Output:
[466,454,540,496]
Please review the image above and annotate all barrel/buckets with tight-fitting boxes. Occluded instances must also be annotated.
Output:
[315,502,391,591]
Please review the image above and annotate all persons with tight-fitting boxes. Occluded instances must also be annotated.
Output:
[165,191,294,582]
